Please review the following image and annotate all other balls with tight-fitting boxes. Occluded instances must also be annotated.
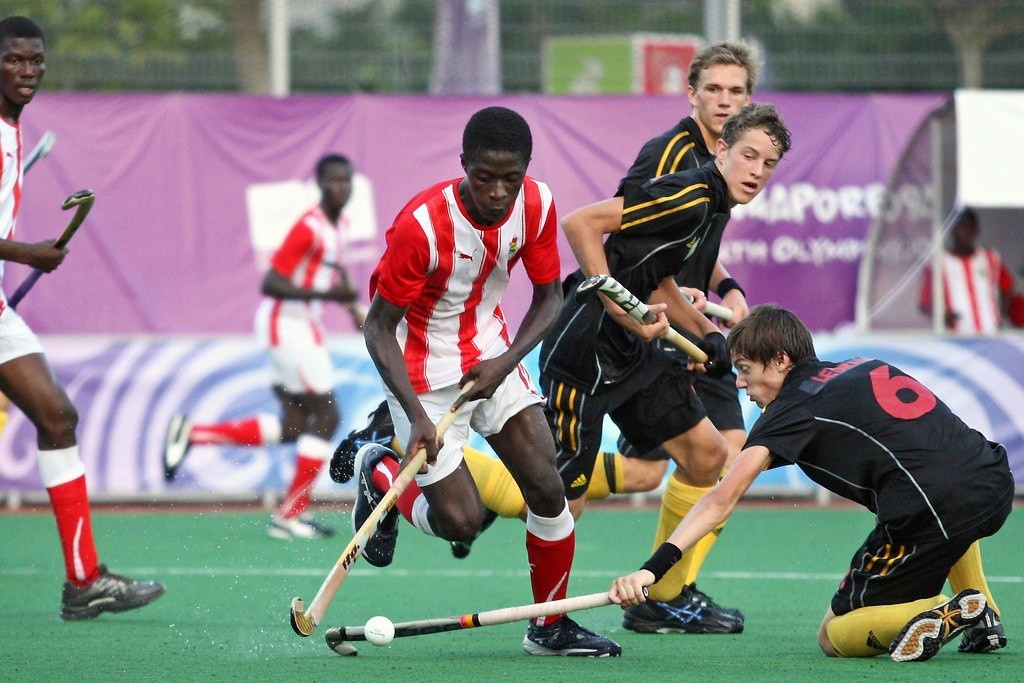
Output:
[364,617,396,646]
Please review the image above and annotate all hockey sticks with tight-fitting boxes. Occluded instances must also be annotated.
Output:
[21,132,58,173]
[574,275,709,361]
[290,377,475,637]
[7,189,96,310]
[681,287,731,321]
[325,259,366,334]
[325,585,648,657]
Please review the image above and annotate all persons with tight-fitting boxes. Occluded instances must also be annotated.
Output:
[915,206,1015,337]
[608,304,1014,663]
[351,106,624,660]
[163,154,367,542]
[329,102,792,637]
[1,14,166,625]
[584,44,757,637]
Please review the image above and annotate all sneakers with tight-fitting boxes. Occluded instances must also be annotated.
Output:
[889,585,986,663]
[522,615,622,658]
[450,504,497,559]
[268,515,332,541]
[163,416,199,479]
[354,444,402,568]
[328,400,396,483]
[60,566,172,621]
[959,606,1009,653]
[622,581,744,634]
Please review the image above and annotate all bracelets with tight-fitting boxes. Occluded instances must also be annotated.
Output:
[717,278,745,300]
[638,542,682,585]
